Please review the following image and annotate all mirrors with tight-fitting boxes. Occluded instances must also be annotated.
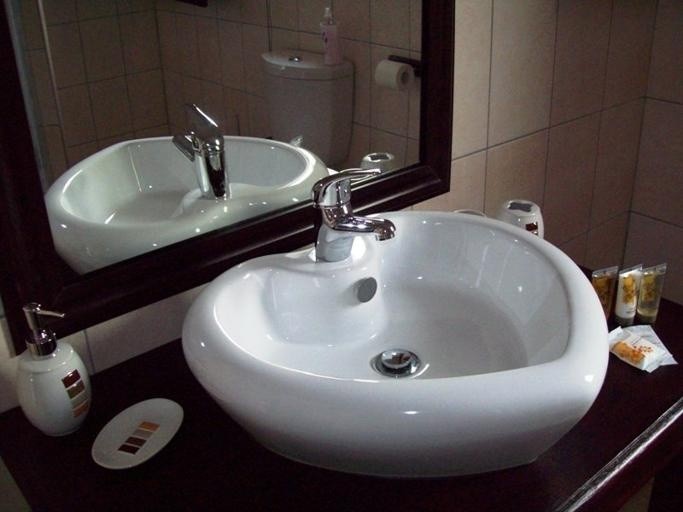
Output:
[0,1,455,359]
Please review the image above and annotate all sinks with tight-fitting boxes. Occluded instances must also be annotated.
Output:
[48,134,329,273]
[180,213,612,480]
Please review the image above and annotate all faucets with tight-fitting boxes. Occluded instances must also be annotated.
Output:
[312,165,394,262]
[173,105,226,198]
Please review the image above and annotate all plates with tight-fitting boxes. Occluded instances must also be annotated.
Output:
[90,397,185,470]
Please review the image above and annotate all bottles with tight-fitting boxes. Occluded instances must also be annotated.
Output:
[319,7,341,66]
